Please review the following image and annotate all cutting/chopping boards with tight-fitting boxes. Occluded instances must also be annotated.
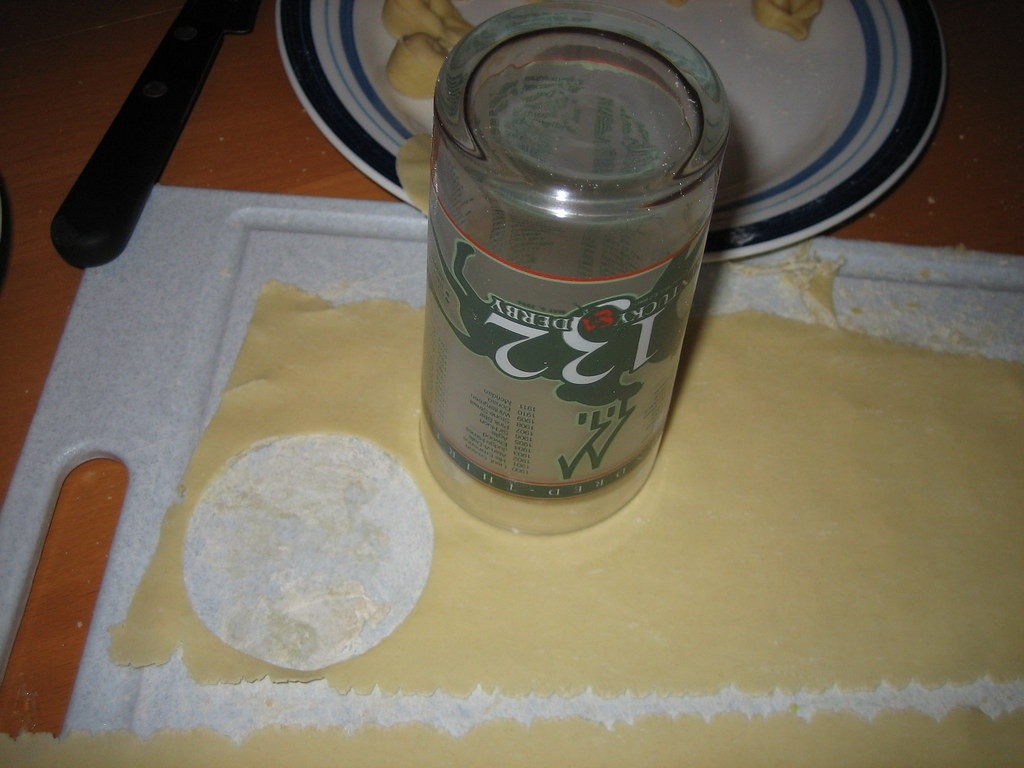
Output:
[1,184,1023,767]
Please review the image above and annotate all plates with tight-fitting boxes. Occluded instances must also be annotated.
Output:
[276,0,946,266]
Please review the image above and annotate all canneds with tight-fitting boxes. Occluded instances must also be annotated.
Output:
[421,1,730,534]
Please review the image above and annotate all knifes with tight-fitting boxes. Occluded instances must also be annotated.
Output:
[50,1,261,268]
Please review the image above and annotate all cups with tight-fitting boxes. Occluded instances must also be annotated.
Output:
[417,0,731,536]
